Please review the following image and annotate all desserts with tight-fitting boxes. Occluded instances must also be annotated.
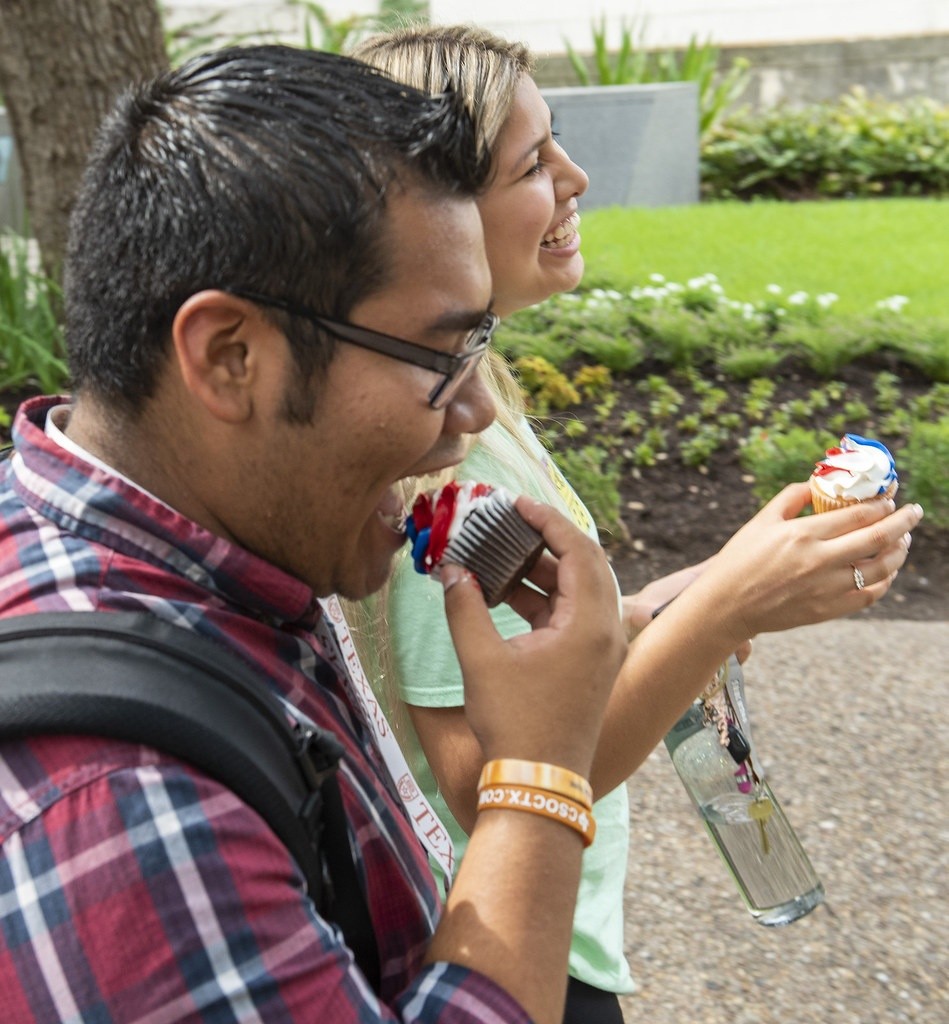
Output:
[808,432,900,514]
[402,479,547,607]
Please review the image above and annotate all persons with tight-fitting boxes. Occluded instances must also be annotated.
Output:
[0,42,629,1024]
[342,22,923,1023]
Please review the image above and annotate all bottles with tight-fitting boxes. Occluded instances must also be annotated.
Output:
[664,703,824,926]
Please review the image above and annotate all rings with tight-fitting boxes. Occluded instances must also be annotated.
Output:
[852,560,864,591]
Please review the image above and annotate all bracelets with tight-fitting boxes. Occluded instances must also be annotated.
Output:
[476,760,597,845]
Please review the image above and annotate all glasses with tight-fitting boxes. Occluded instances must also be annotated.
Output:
[128,279,500,410]
